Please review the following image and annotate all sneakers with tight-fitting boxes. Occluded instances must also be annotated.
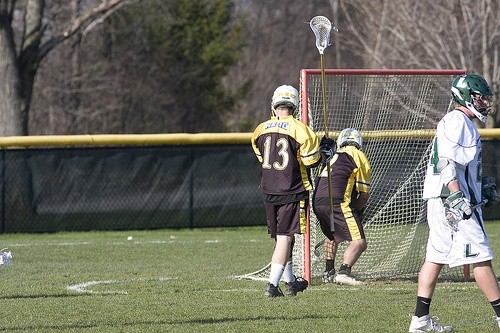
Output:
[409,314,452,333]
[321,273,335,283]
[497,317,500,328]
[336,274,366,286]
[285,275,308,296]
[264,282,285,297]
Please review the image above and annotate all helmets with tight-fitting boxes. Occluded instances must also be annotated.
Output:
[271,85,299,110]
[337,128,362,150]
[451,74,495,122]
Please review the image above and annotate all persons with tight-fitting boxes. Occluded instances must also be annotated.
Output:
[251,84,336,297]
[410,73,500,333]
[312,127,373,285]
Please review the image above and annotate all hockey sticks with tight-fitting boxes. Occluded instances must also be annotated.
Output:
[309,16,335,231]
[443,199,488,230]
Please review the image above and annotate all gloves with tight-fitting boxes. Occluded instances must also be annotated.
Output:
[482,176,498,209]
[446,191,472,220]
[357,205,366,222]
[320,135,338,162]
